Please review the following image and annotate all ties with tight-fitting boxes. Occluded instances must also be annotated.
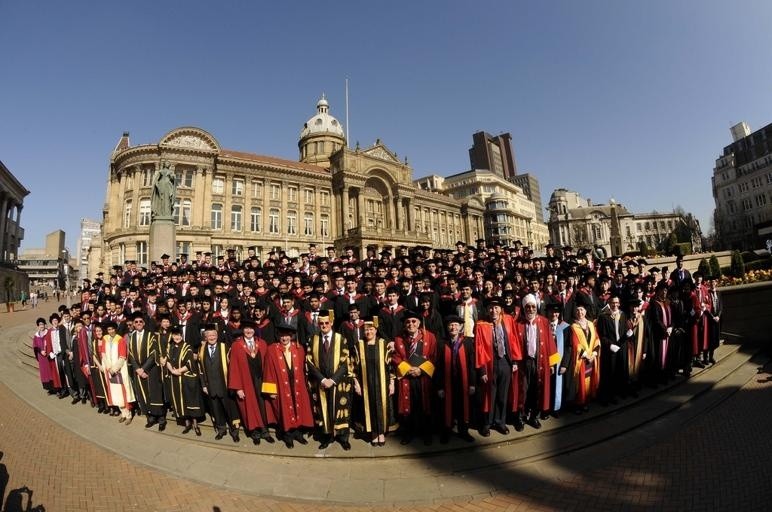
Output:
[392,308,393,312]
[409,336,415,356]
[322,334,330,351]
[137,333,141,359]
[210,345,215,356]
[527,320,536,358]
[493,319,505,359]
[248,340,254,353]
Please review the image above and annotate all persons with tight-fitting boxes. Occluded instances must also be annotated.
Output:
[5,237,727,450]
[150,160,176,216]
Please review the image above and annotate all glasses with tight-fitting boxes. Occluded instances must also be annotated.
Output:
[407,319,416,324]
[319,321,329,325]
[135,320,143,324]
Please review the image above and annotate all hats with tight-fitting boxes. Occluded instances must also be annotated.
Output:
[203,321,219,330]
[546,302,563,312]
[155,294,185,332]
[692,270,719,280]
[164,262,187,294]
[161,247,259,261]
[84,260,163,296]
[185,264,268,309]
[304,290,326,305]
[476,237,542,295]
[523,293,537,308]
[359,315,382,330]
[239,318,255,328]
[343,244,413,284]
[274,323,300,348]
[79,309,117,329]
[268,244,342,287]
[414,241,476,288]
[387,285,404,294]
[544,243,623,283]
[282,293,296,305]
[312,308,336,323]
[623,257,669,273]
[36,304,68,325]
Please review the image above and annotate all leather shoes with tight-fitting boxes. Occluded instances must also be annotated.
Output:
[47,388,95,407]
[540,405,589,419]
[395,430,475,445]
[480,414,542,435]
[602,379,658,406]
[182,424,192,434]
[253,435,307,449]
[378,434,386,447]
[319,439,335,448]
[696,357,715,369]
[194,425,201,435]
[159,421,167,431]
[371,433,378,446]
[214,429,226,439]
[144,419,158,428]
[98,403,140,424]
[230,432,240,442]
[336,436,351,450]
